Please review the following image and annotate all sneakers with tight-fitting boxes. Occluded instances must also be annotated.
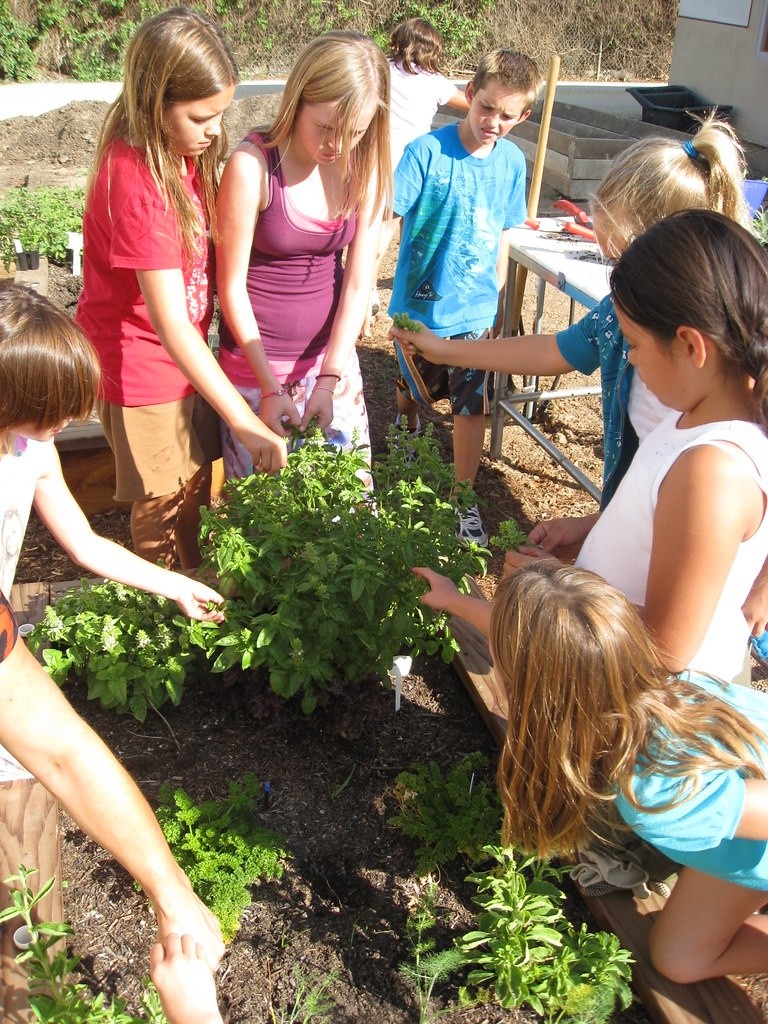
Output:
[385,413,421,466]
[448,497,489,550]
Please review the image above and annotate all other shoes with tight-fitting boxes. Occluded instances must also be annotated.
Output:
[369,286,380,328]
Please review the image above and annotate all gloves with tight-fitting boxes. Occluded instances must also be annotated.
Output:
[570,851,671,899]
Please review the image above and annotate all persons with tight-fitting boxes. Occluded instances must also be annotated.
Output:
[0,279,224,624]
[76,7,288,569]
[150,934,224,1024]
[215,29,393,523]
[370,17,768,684]
[411,558,768,983]
[0,592,225,974]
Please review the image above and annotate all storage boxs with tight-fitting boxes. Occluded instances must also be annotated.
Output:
[624,84,734,132]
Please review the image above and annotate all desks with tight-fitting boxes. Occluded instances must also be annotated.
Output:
[493,216,615,507]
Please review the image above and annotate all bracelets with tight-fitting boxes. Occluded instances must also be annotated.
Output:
[315,373,341,382]
[313,387,334,395]
[259,390,288,398]
